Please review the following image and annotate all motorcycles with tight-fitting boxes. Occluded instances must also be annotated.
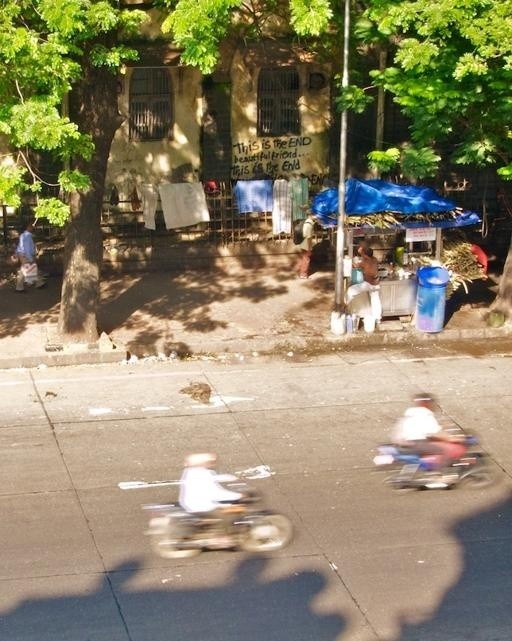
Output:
[372,429,501,493]
[144,491,295,563]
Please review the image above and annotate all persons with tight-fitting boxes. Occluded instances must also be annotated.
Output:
[15,223,47,292]
[292,208,317,279]
[390,391,470,459]
[342,239,383,326]
[177,452,253,515]
